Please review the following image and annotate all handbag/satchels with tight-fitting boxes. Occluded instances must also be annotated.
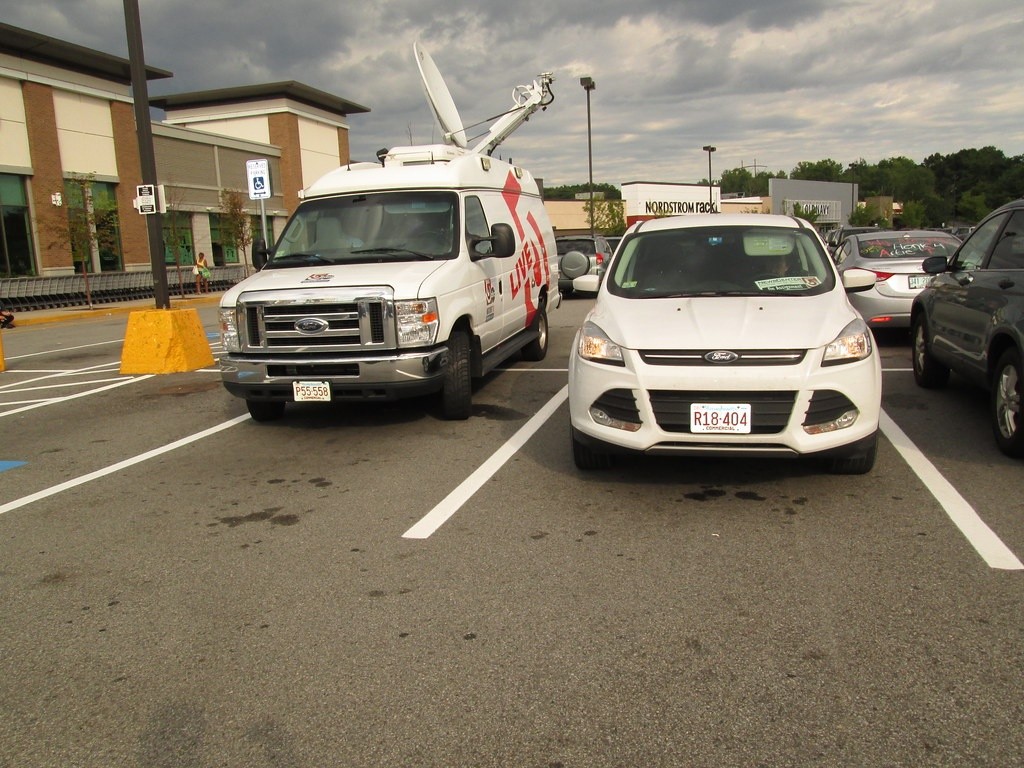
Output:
[192,264,199,275]
[201,266,211,278]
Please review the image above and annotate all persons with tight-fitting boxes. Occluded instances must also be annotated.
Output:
[0,306,15,329]
[191,252,209,295]
[766,249,815,296]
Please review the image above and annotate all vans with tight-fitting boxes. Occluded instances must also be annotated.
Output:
[216,145,558,417]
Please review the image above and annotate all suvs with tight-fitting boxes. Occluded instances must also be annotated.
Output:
[826,224,881,258]
[554,235,613,293]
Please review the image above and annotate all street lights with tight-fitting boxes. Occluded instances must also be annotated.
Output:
[579,77,596,237]
[701,145,716,212]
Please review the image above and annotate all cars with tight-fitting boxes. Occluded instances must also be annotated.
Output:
[569,213,881,474]
[831,231,984,345]
[911,197,1024,444]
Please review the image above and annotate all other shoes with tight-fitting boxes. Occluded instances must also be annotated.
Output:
[198,292,202,295]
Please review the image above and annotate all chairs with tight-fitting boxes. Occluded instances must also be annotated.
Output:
[308,216,352,253]
